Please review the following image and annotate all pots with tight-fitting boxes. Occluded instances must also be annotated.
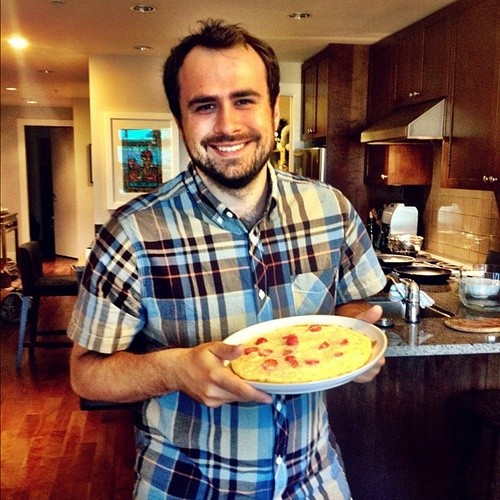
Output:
[376,254,415,266]
[392,266,452,286]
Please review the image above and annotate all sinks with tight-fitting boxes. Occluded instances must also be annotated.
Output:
[366,296,455,320]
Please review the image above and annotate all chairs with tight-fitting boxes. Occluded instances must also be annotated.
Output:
[15,241,80,377]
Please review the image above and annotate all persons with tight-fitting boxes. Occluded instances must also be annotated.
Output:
[69,18,389,500]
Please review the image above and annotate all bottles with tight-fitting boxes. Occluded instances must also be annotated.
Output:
[366,211,378,250]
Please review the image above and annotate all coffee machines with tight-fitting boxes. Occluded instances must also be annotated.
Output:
[379,203,419,253]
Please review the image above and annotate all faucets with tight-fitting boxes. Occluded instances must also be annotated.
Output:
[386,271,420,324]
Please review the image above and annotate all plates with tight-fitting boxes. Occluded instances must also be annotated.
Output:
[221,315,388,395]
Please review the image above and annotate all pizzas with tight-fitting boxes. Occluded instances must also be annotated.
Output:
[230,323,373,383]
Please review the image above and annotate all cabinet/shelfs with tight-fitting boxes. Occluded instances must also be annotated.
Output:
[440,0,500,190]
[364,144,432,185]
[367,32,395,122]
[301,43,369,140]
[393,4,448,109]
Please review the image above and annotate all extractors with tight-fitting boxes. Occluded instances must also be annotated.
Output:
[360,97,444,144]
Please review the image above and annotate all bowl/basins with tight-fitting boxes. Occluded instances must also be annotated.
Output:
[458,271,500,312]
[387,234,423,254]
[461,277,500,299]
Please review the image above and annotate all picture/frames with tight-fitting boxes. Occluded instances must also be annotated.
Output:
[103,111,181,209]
